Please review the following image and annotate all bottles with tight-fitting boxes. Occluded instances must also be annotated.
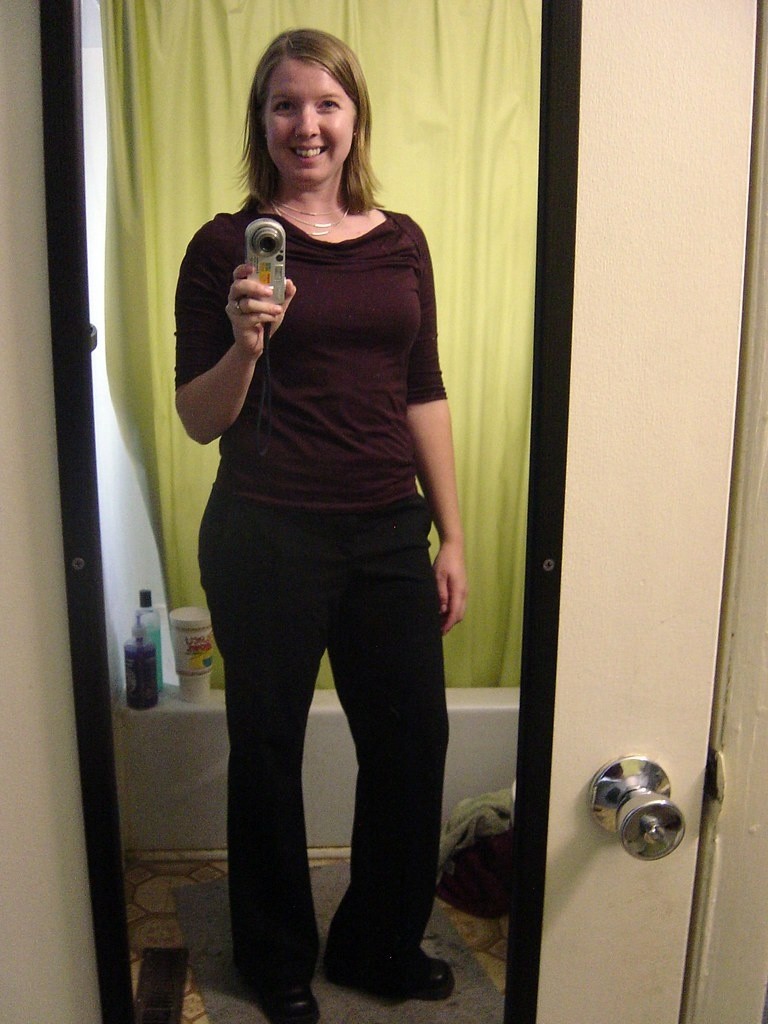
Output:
[132,589,163,692]
[124,615,158,709]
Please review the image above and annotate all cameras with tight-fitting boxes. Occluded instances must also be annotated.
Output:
[244,218,284,304]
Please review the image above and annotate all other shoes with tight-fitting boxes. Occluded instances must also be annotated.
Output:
[254,979,320,1024]
[325,956,454,1001]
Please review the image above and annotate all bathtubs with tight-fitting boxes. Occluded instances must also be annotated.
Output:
[115,677,519,850]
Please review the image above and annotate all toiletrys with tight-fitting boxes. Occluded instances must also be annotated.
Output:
[121,588,162,708]
[170,607,214,702]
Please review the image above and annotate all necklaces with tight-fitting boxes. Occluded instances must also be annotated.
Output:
[270,199,350,236]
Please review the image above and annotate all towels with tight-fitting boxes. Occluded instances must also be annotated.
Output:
[431,789,515,918]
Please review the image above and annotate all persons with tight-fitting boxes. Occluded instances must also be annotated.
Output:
[174,29,472,1024]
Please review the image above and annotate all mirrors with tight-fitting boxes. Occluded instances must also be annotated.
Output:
[40,1,581,1024]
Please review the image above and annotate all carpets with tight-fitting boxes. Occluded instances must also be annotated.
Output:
[170,863,505,1024]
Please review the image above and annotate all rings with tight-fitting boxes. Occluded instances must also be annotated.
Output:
[235,299,243,314]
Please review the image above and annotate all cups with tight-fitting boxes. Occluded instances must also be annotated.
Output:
[169,606,213,703]
